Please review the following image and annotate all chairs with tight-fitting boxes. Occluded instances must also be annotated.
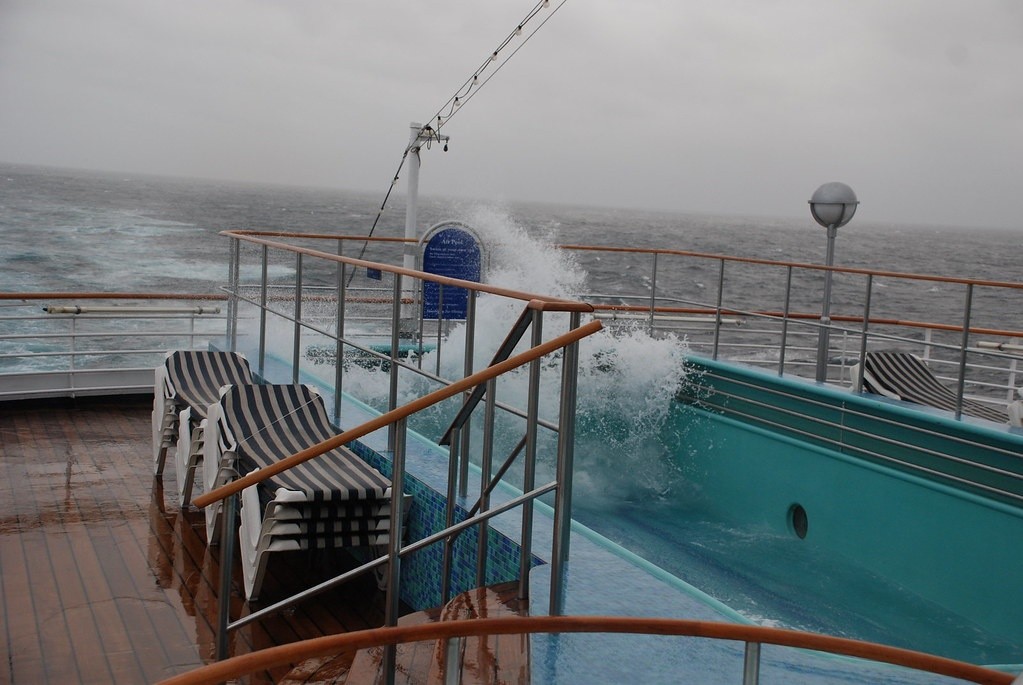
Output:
[151,351,413,602]
[850,352,1010,425]
